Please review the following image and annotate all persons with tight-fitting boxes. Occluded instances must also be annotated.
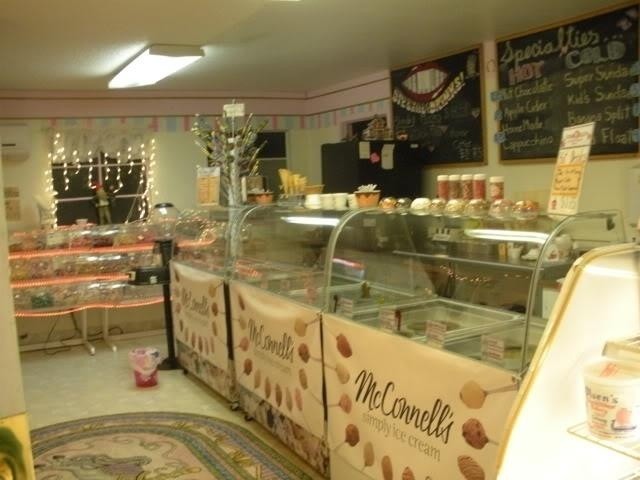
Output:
[91,182,115,225]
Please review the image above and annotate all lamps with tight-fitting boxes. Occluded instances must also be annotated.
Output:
[106,45,204,89]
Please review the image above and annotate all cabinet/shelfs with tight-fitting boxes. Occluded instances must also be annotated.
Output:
[8,219,170,359]
[170,202,303,410]
[324,204,625,478]
[229,203,435,477]
[493,240,640,480]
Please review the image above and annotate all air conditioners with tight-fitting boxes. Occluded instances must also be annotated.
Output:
[0,125,32,162]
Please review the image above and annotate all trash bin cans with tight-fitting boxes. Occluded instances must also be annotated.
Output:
[128,347,159,387]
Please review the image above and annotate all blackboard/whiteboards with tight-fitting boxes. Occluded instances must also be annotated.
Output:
[390,43,488,170]
[494,0,640,166]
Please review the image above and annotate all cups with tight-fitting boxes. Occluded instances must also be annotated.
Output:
[305,191,359,211]
[437,174,504,200]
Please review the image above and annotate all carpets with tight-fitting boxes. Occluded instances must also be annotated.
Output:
[30,412,313,480]
